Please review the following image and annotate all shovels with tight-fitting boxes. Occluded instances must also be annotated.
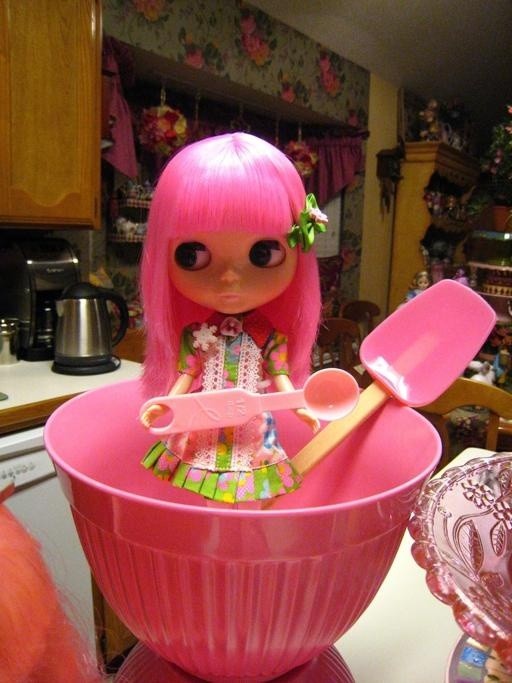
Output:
[261,279,497,509]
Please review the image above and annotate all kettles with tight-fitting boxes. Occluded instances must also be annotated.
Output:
[51,281,129,375]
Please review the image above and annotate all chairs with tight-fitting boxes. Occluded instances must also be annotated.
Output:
[319,298,381,370]
[412,378,511,474]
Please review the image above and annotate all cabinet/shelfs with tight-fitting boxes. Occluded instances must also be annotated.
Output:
[385,141,482,315]
[0,0,105,231]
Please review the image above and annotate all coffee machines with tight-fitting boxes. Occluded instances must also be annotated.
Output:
[16,237,81,362]
